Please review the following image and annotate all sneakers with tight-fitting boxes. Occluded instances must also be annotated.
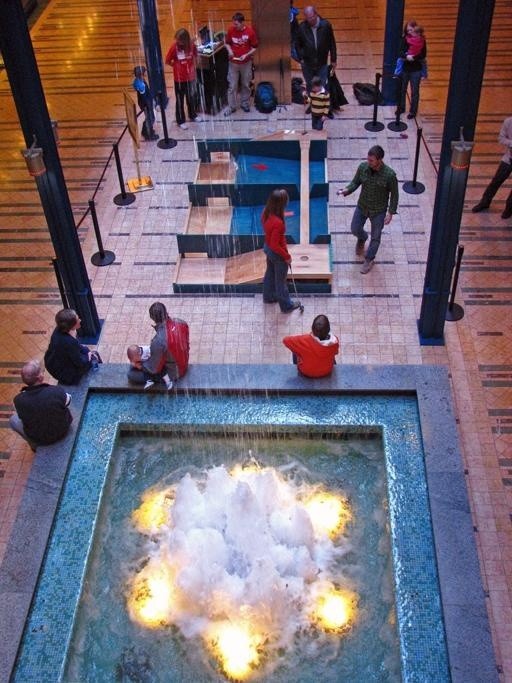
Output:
[281,301,300,312]
[395,109,404,115]
[408,113,415,119]
[167,380,173,390]
[224,106,236,116]
[473,203,488,212]
[356,233,368,255]
[360,258,375,274]
[502,209,511,218]
[141,129,158,140]
[177,123,187,130]
[189,116,201,122]
[240,104,250,112]
[144,381,154,390]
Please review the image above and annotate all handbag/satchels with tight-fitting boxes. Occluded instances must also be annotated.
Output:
[354,82,384,105]
[254,82,278,112]
[291,77,304,105]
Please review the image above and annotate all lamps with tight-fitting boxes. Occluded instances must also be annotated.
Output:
[20,130,48,177]
[449,123,474,170]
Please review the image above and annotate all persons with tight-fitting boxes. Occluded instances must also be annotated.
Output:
[132,66,159,140]
[337,145,399,274]
[44,309,102,384]
[283,315,339,378]
[9,358,71,453]
[392,26,427,81]
[262,189,301,312]
[472,117,512,219]
[395,20,426,119]
[127,345,174,390]
[127,301,190,383]
[295,6,337,119]
[306,76,330,130]
[166,12,259,130]
